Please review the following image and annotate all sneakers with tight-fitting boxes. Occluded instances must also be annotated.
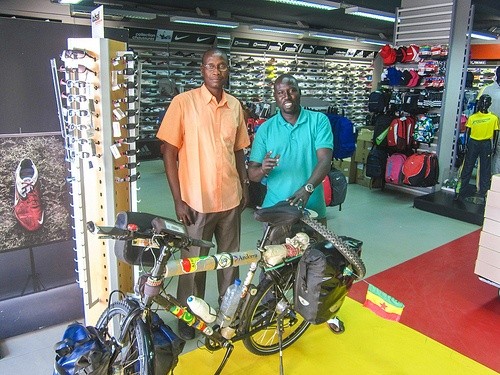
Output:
[13,157,48,232]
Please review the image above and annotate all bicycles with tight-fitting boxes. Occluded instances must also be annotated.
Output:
[85,212,366,375]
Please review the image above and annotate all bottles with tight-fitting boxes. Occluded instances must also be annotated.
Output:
[186,296,218,323]
[214,280,242,327]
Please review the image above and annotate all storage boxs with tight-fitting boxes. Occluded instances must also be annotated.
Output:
[332,127,380,188]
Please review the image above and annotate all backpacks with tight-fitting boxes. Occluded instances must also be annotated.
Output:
[361,91,443,190]
[328,112,358,161]
[322,169,348,207]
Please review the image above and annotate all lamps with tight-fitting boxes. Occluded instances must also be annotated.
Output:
[70,4,157,21]
[344,6,395,23]
[248,24,305,36]
[466,29,498,40]
[271,0,341,10]
[309,31,357,42]
[361,39,388,46]
[169,16,239,30]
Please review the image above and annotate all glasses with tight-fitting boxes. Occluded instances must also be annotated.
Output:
[203,63,229,72]
[114,52,142,186]
[59,47,103,164]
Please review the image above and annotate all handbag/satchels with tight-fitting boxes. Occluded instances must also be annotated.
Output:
[292,235,365,325]
[52,321,112,375]
[131,311,185,375]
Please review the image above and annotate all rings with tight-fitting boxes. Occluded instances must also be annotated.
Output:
[179,219,183,222]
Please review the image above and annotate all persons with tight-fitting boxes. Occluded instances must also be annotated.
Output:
[162,49,250,351]
[452,94,499,203]
[248,74,346,335]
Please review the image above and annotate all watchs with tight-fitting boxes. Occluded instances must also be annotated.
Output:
[302,183,315,194]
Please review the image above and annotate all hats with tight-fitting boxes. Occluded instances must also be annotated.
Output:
[377,44,419,90]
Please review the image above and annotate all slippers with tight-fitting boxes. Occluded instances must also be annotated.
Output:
[327,315,346,335]
